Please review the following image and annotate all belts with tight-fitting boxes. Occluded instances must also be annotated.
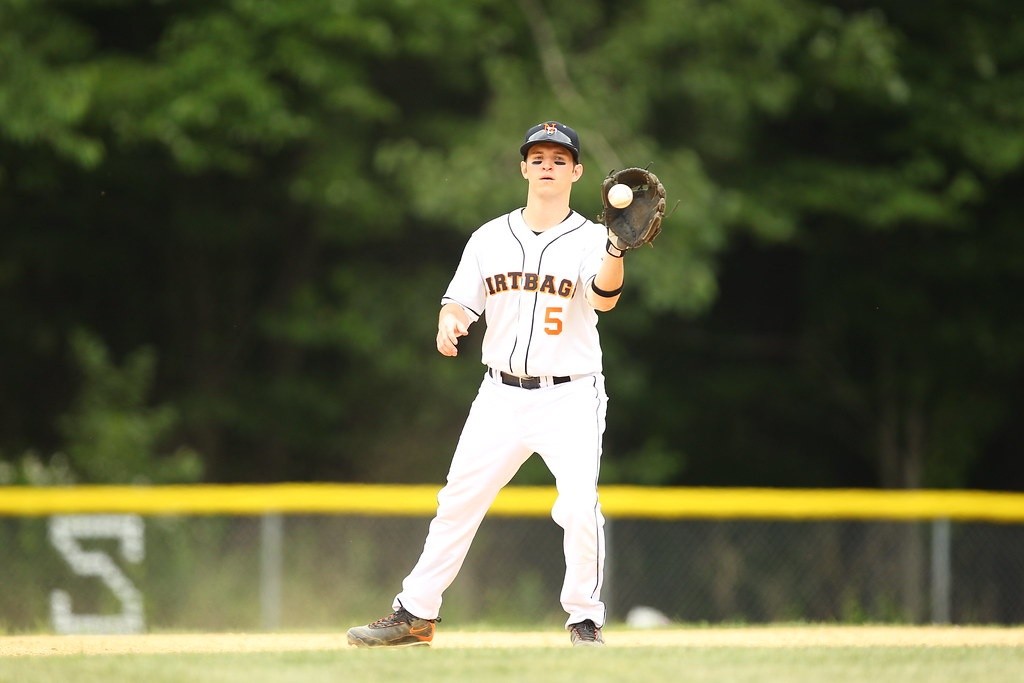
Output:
[489,367,571,390]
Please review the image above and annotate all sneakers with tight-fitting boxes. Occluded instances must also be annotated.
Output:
[347,598,442,650]
[569,619,604,647]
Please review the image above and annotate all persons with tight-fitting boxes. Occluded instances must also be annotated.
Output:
[346,122,625,648]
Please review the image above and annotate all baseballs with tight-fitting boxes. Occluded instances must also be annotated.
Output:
[607,183,634,209]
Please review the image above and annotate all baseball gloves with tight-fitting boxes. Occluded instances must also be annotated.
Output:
[600,166,668,258]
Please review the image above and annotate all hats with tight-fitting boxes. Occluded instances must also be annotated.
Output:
[520,121,580,164]
